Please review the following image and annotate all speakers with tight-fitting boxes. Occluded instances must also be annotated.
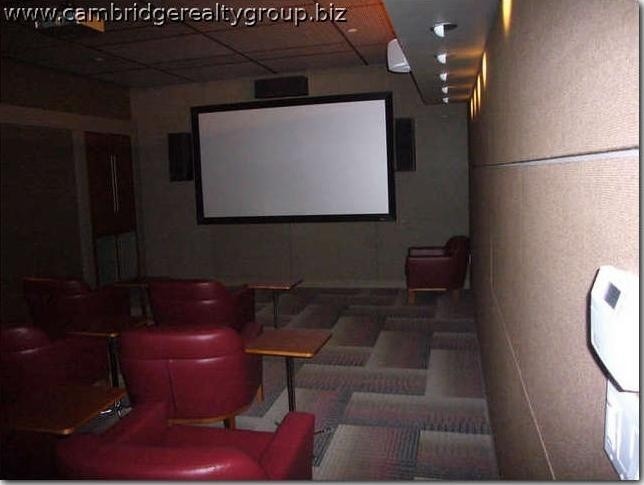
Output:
[254,75,309,99]
[394,117,416,172]
[168,132,193,181]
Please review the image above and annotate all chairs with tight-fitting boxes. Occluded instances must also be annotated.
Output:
[0,276,315,480]
[405,235,470,305]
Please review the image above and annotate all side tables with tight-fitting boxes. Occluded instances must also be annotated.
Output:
[245,330,333,458]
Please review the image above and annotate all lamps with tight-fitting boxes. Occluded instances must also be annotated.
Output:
[387,37,412,74]
